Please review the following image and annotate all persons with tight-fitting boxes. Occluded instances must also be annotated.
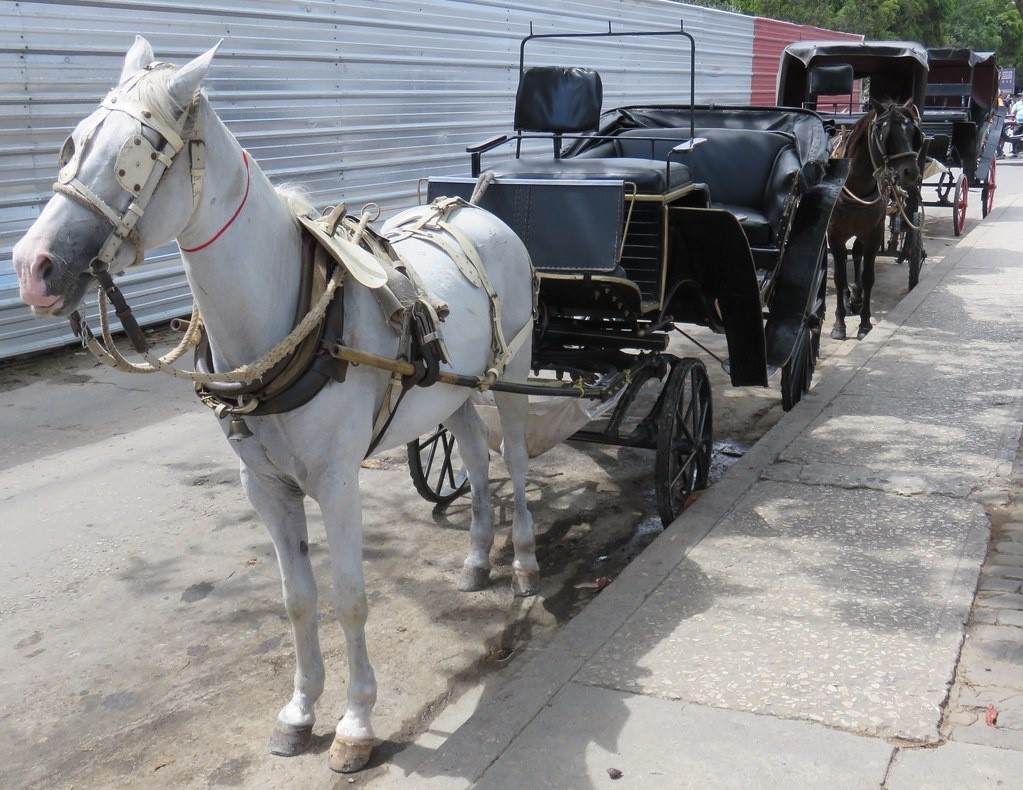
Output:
[993,91,1012,160]
[1012,90,1023,159]
[1008,92,1022,157]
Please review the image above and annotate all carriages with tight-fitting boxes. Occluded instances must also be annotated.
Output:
[10,20,1007,774]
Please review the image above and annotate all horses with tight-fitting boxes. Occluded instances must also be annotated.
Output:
[827,95,921,341]
[13,32,542,774]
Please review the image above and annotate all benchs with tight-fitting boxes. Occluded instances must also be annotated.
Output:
[801,61,865,133]
[565,127,804,271]
[468,65,707,207]
[921,82,973,120]
